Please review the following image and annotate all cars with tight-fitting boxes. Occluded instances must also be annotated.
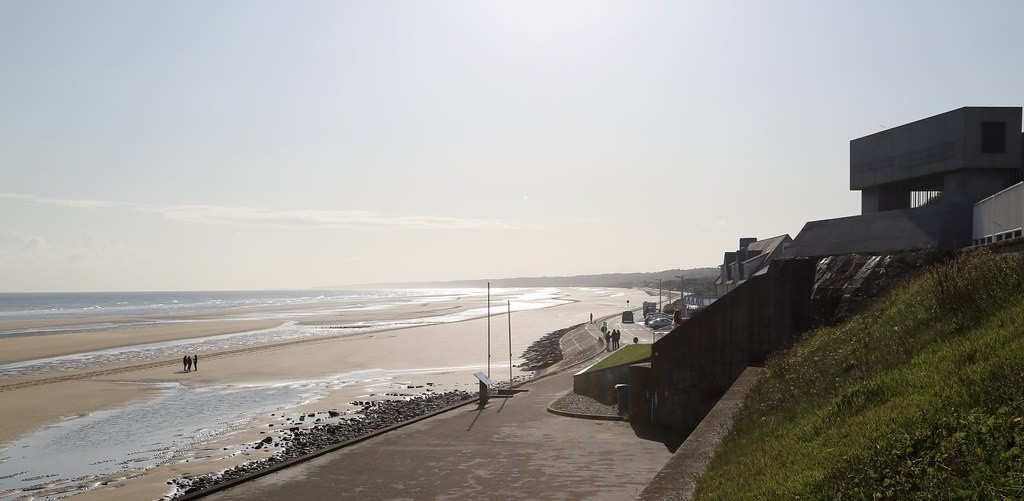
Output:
[649,319,673,329]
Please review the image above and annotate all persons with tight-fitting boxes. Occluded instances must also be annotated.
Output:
[183,355,192,371]
[606,329,621,352]
[193,355,198,371]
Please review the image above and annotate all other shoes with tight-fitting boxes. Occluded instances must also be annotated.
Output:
[613,350,615,351]
[194,370,197,371]
[615,349,617,350]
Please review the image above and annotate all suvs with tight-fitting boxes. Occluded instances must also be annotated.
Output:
[645,312,673,325]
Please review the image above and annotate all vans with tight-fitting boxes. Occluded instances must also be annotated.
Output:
[622,311,633,323]
